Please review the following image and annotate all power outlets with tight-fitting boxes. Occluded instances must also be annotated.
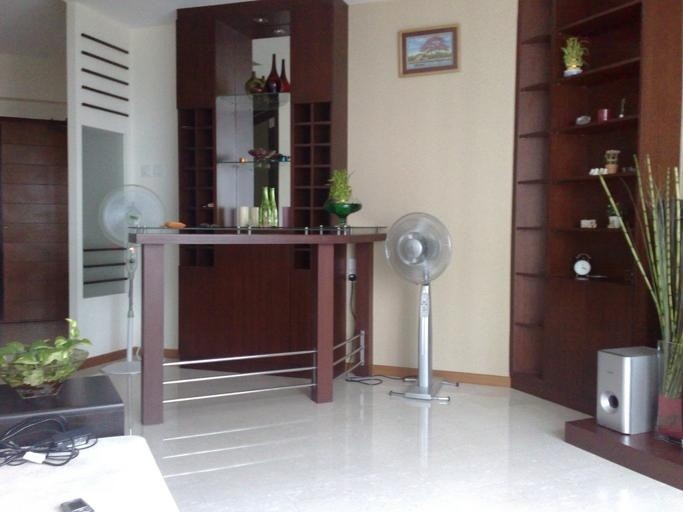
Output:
[346,258,358,285]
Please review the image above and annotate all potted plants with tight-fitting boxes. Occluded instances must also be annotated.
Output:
[559,33,591,78]
[595,151,683,447]
[323,167,362,228]
[0,316,94,401]
[606,197,630,230]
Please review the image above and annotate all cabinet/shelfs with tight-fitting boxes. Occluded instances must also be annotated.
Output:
[175,0,349,379]
[509,0,683,418]
[1,375,124,452]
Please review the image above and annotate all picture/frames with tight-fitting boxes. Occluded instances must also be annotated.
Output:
[397,22,461,79]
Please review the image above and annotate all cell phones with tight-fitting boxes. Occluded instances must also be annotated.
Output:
[60,498,94,512]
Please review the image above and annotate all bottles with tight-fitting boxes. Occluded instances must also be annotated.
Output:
[244,71,263,93]
[264,54,280,92]
[280,58,290,92]
[269,187,278,226]
[258,186,272,226]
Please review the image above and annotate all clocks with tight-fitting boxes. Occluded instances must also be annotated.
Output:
[572,251,593,280]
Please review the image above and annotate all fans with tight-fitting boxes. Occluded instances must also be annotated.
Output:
[97,185,167,375]
[382,211,459,401]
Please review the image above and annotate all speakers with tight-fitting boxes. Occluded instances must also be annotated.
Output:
[594,345,660,435]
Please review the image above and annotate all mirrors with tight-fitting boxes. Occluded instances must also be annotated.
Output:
[214,6,294,230]
